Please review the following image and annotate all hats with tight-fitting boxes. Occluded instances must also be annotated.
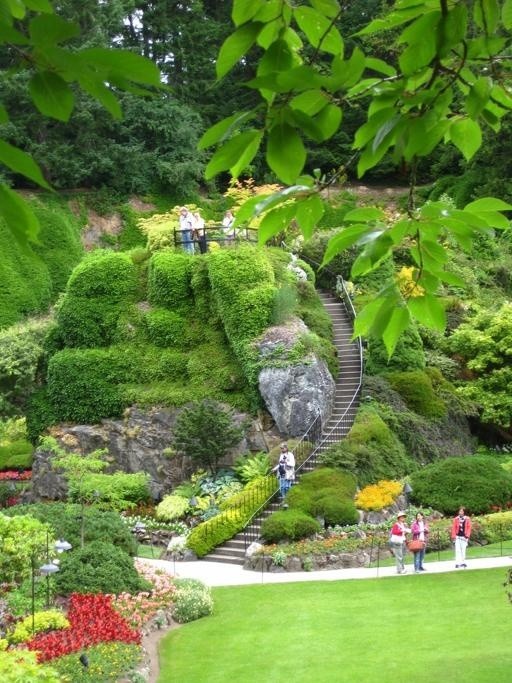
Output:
[396,511,408,518]
[280,443,287,449]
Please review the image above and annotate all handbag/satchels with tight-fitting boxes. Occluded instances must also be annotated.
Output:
[408,540,426,552]
[390,534,404,545]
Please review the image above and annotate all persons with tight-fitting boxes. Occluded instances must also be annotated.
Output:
[179,207,205,253]
[411,511,431,573]
[222,211,236,244]
[271,443,295,498]
[390,512,411,574]
[452,506,471,568]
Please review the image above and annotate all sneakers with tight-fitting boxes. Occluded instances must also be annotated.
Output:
[398,563,467,574]
